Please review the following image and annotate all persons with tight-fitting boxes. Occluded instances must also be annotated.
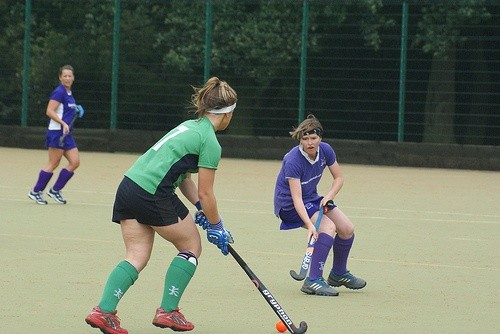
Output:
[274,114,367,297]
[84,76,238,334]
[28,65,85,205]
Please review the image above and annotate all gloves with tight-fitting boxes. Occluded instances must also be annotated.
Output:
[76,105,84,118]
[207,219,234,256]
[194,201,207,230]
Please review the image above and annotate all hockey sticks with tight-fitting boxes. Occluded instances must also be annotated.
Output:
[290,206,326,281]
[200,211,308,334]
[59,114,79,145]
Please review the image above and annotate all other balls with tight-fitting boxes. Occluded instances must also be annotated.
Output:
[276,320,287,332]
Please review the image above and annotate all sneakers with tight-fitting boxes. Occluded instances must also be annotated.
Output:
[152,307,194,332]
[85,306,128,334]
[300,277,339,296]
[28,190,47,205]
[327,269,367,290]
[46,188,67,204]
